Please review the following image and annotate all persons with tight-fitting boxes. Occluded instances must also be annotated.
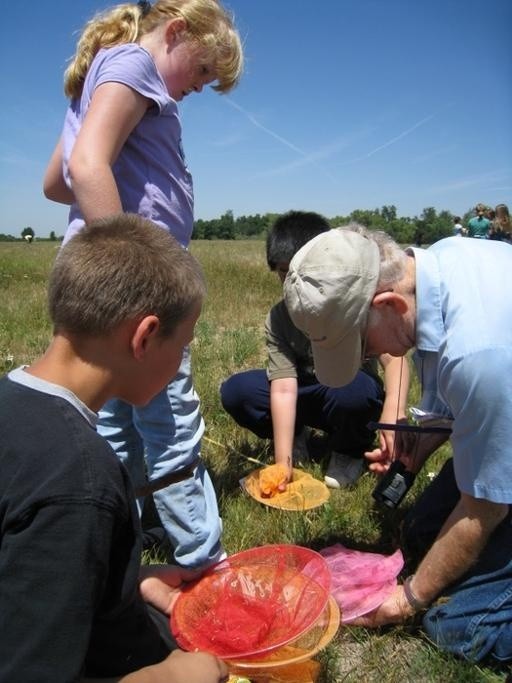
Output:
[467,203,493,238]
[221,211,416,489]
[38,0,247,567]
[283,221,512,666]
[490,204,512,244]
[484,207,495,220]
[0,212,230,683]
[453,215,467,234]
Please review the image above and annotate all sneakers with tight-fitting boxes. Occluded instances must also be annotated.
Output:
[291,425,312,468]
[324,452,363,489]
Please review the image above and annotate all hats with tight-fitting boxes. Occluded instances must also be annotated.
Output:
[283,226,379,387]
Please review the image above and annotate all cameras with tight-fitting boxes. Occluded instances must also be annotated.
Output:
[370,459,416,510]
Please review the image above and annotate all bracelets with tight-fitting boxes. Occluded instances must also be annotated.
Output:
[404,573,429,614]
